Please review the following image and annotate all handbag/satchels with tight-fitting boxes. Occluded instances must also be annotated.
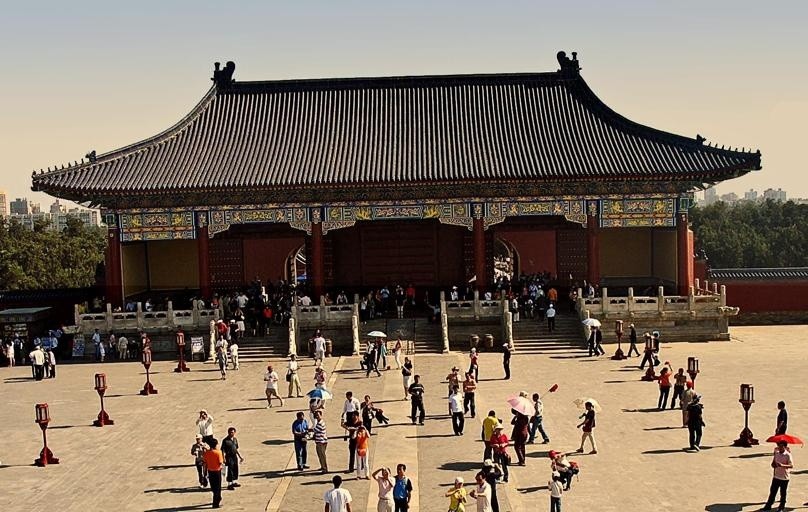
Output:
[357,449,365,456]
[286,369,295,381]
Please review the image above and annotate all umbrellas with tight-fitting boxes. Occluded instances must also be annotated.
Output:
[766,434,804,444]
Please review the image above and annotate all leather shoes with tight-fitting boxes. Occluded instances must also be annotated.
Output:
[198,482,241,508]
[297,465,370,480]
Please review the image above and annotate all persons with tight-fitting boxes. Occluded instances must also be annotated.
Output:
[2,269,705,511]
[775,401,787,436]
[760,440,793,512]
[774,442,791,456]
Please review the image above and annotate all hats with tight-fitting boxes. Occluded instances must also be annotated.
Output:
[196,434,202,439]
[483,459,495,468]
[494,423,503,429]
[455,477,463,484]
[686,382,691,388]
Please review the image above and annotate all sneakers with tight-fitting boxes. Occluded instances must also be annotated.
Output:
[693,444,699,451]
[500,440,597,491]
[264,395,305,409]
[404,397,475,436]
